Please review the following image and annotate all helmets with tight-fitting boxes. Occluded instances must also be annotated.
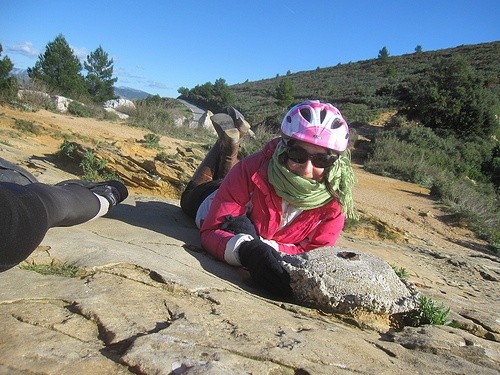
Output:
[281,99,349,152]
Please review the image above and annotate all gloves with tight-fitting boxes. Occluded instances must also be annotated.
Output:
[220,214,259,240]
[238,238,292,285]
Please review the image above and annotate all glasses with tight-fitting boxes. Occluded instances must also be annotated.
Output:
[281,139,338,168]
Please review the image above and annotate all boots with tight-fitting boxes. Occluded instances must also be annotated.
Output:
[190,106,250,186]
[210,112,241,180]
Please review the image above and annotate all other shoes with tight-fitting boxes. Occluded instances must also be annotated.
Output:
[56,179,129,213]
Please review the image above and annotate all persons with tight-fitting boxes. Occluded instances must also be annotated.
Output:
[0,157,129,274]
[180,99,356,296]
